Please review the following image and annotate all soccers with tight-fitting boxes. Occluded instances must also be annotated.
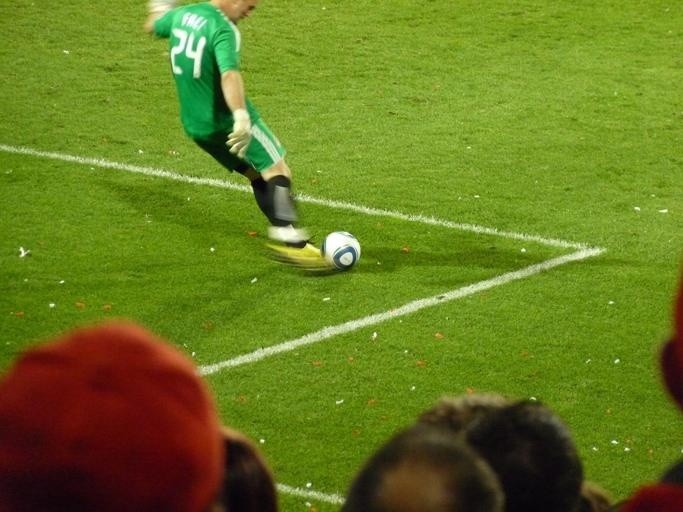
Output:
[321,230,361,272]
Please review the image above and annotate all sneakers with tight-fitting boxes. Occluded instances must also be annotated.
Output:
[267,238,329,272]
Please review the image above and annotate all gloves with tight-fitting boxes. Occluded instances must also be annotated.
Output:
[227,114,252,160]
[144,0,175,17]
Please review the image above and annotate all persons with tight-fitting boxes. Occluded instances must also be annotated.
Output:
[145,1,339,272]
[341,425,504,512]
[417,393,609,509]
[466,400,582,512]
[608,282,683,512]
[219,432,279,512]
